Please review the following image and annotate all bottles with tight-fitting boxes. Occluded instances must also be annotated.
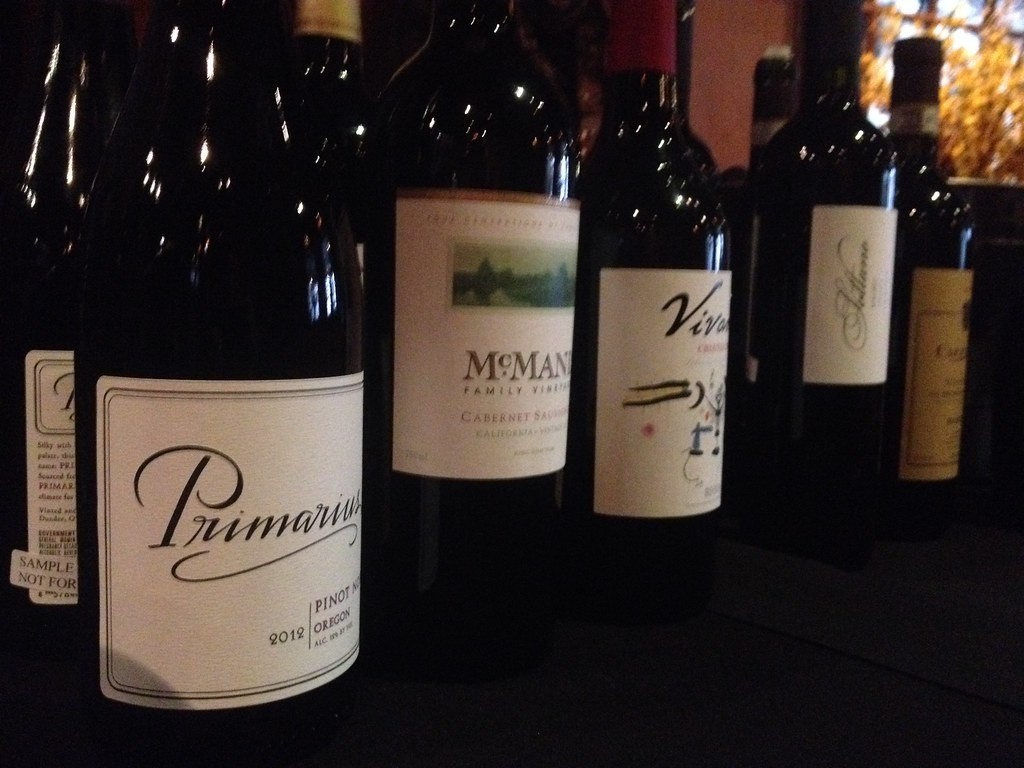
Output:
[0,0,1024,768]
